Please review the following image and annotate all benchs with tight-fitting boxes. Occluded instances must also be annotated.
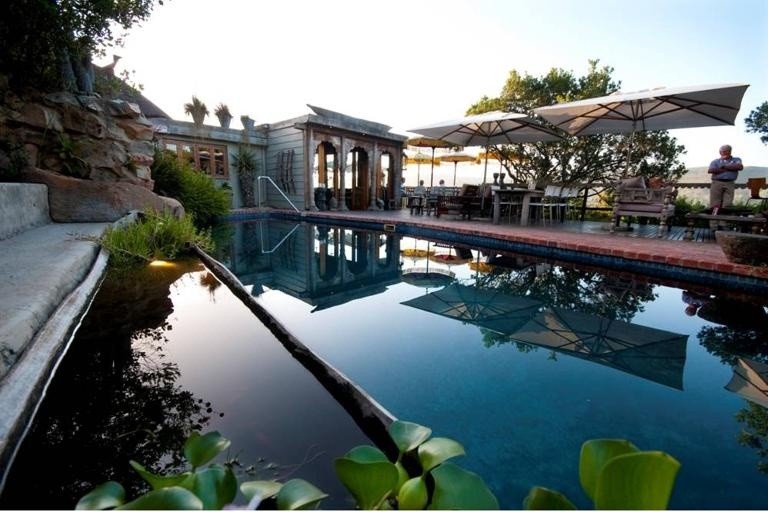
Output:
[607,182,681,238]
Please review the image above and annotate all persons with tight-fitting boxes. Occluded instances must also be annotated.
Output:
[708,145,745,234]
[439,180,446,188]
[414,180,428,215]
[401,177,413,197]
[680,288,714,317]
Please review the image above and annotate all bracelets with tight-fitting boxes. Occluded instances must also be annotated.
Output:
[720,165,725,170]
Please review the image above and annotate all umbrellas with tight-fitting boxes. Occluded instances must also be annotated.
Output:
[507,273,690,392]
[397,244,546,339]
[403,134,519,187]
[532,81,752,180]
[397,236,510,289]
[406,108,566,211]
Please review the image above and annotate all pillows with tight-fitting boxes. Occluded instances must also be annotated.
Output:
[645,175,664,197]
[621,176,648,198]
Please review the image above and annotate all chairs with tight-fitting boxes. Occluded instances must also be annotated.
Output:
[403,182,578,225]
